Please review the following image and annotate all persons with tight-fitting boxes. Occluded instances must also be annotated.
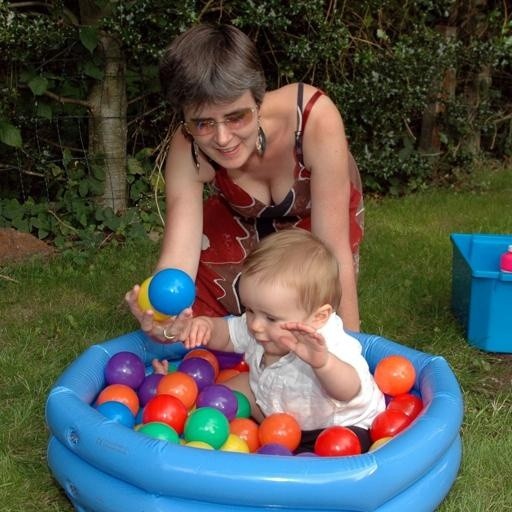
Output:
[183,226,386,456]
[122,20,365,345]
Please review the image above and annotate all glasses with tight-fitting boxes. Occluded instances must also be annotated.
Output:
[181,108,258,136]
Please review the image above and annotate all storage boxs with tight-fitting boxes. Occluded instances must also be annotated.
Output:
[446,230,512,356]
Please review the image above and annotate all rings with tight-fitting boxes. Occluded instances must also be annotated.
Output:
[162,325,176,340]
